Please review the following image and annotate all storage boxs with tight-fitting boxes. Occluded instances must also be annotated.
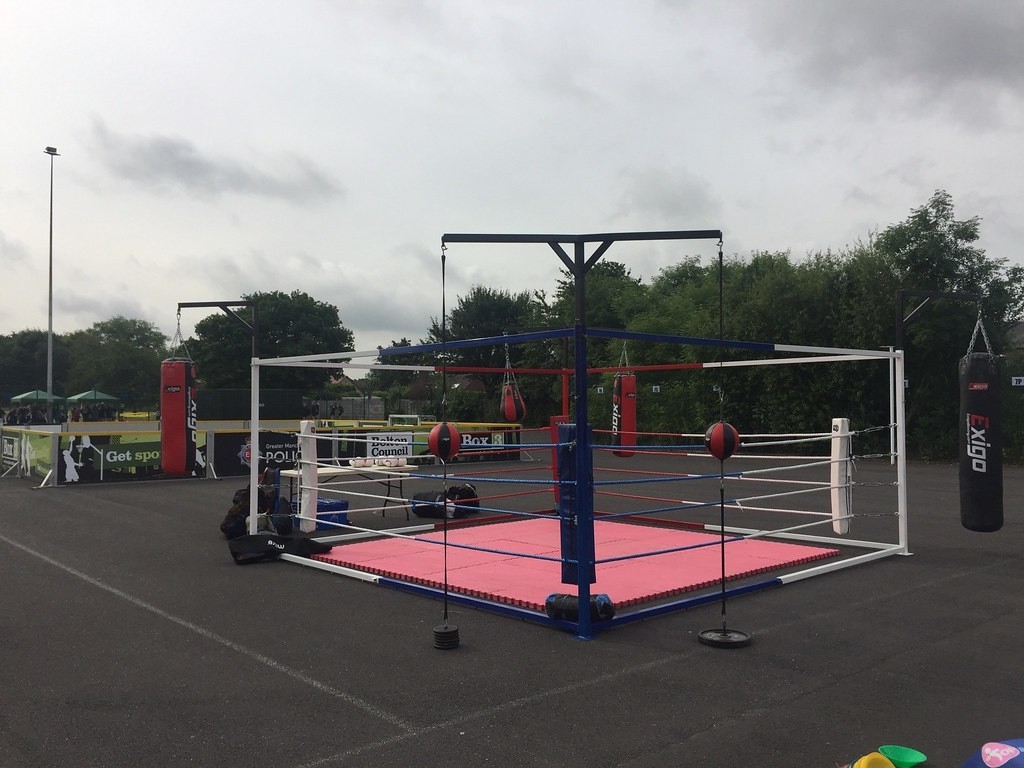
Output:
[290,498,350,531]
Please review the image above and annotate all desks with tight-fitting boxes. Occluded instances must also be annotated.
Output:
[279,464,419,522]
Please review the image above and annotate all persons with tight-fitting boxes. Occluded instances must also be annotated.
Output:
[3,400,138,425]
[330,402,337,419]
[154,400,161,421]
[337,403,344,419]
[303,400,313,420]
[312,400,319,419]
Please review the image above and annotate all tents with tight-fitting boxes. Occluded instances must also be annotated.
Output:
[65,389,120,414]
[10,390,65,418]
